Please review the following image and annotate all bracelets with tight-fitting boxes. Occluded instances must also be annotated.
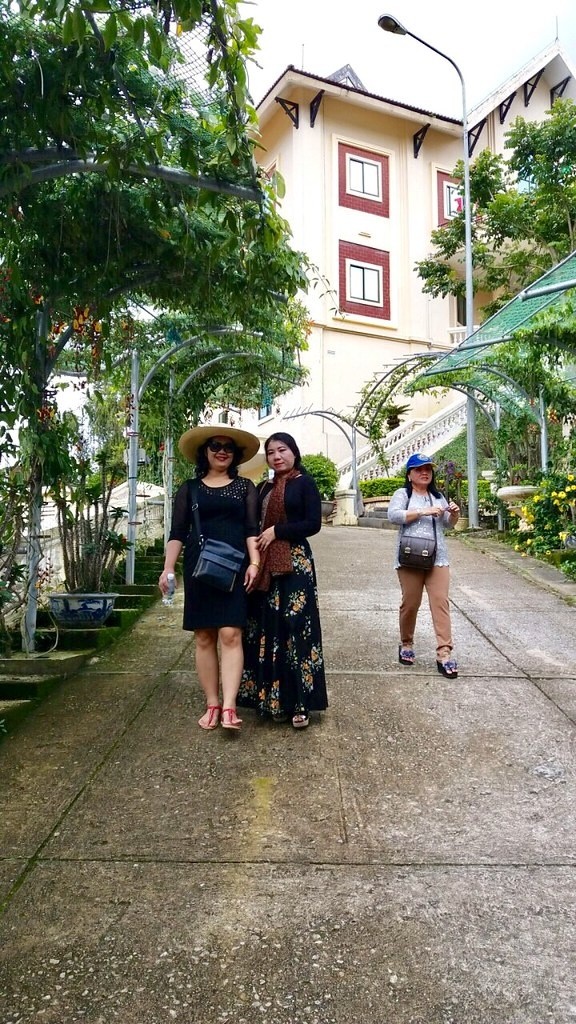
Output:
[416,508,421,520]
[451,512,460,520]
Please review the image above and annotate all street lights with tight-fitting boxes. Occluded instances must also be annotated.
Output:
[377,13,477,532]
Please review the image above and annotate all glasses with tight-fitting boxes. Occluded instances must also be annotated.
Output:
[208,440,237,454]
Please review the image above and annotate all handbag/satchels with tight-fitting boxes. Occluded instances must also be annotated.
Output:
[398,535,438,571]
[189,537,247,594]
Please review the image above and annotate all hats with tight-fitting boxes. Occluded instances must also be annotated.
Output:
[406,453,439,469]
[178,422,260,465]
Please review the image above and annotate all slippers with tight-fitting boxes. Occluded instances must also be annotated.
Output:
[398,644,416,666]
[272,714,288,722]
[292,713,309,727]
[198,705,222,730]
[219,708,242,730]
[435,658,459,679]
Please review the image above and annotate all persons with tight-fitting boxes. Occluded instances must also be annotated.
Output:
[235,432,330,729]
[159,422,262,732]
[387,453,462,680]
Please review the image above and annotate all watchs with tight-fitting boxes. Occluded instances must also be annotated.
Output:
[250,559,262,570]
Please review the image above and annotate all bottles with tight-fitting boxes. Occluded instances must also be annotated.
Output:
[161,573,177,607]
